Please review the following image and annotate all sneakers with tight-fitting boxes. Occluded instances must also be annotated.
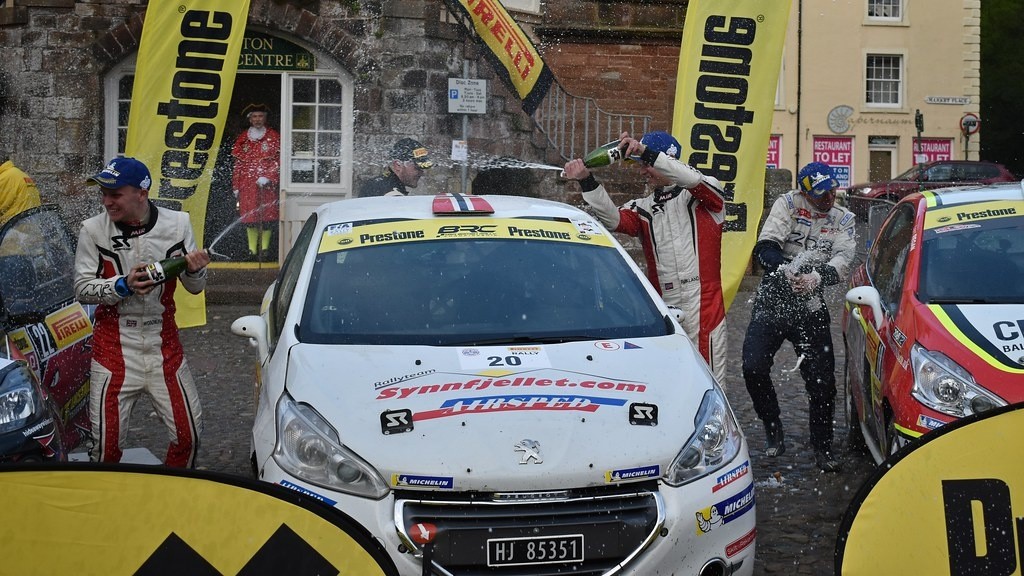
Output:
[814,448,838,472]
[766,445,786,458]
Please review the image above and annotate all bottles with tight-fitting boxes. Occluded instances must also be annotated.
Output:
[793,272,826,319]
[563,139,632,174]
[137,249,210,289]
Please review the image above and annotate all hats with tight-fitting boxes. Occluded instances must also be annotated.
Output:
[85,157,152,191]
[624,132,681,164]
[389,139,434,169]
[241,99,273,117]
[797,162,840,200]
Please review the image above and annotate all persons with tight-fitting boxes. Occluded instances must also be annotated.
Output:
[73,156,214,468]
[360,138,432,197]
[0,139,43,255]
[561,132,729,401]
[742,163,857,472]
[231,99,282,262]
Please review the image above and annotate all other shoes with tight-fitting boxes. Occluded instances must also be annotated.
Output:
[261,249,273,263]
[241,250,259,262]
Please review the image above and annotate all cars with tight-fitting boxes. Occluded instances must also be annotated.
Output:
[844,160,1018,220]
[840,179,1024,468]
[0,202,94,466]
[228,191,757,576]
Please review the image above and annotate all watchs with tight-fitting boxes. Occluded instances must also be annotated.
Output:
[115,276,133,298]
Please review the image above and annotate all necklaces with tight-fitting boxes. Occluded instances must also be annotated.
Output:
[125,203,150,225]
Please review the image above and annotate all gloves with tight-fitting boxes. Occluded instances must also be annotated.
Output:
[233,189,239,198]
[256,177,270,188]
